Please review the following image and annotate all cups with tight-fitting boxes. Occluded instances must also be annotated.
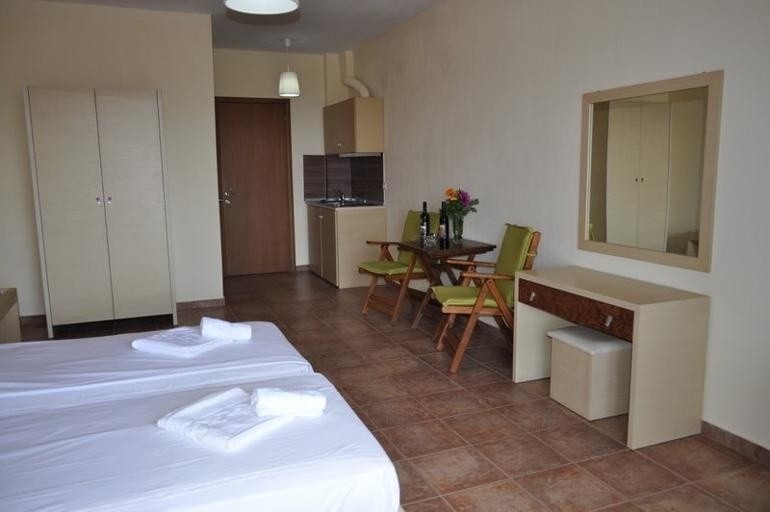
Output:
[427,233,438,246]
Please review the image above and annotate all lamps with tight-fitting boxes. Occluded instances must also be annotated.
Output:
[278,37,302,99]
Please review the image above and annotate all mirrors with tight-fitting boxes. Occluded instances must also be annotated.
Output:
[577,67,727,273]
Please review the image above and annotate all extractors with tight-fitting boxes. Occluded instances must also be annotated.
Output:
[337,153,382,159]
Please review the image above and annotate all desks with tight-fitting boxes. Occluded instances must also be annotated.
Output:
[512,264,713,449]
[397,238,499,342]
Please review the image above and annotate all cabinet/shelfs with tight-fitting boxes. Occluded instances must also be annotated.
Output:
[605,95,673,256]
[306,201,389,290]
[323,96,386,156]
[22,80,180,337]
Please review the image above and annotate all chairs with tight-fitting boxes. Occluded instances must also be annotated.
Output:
[428,221,541,371]
[356,208,442,326]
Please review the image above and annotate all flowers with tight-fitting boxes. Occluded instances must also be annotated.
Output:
[442,186,480,239]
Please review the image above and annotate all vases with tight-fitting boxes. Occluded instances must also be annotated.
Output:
[451,215,465,244]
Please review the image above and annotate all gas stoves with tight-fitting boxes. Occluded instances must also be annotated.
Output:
[309,194,382,207]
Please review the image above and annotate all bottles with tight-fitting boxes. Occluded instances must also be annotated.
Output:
[420,202,430,244]
[439,202,450,248]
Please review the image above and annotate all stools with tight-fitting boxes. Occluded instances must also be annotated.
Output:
[545,323,634,420]
